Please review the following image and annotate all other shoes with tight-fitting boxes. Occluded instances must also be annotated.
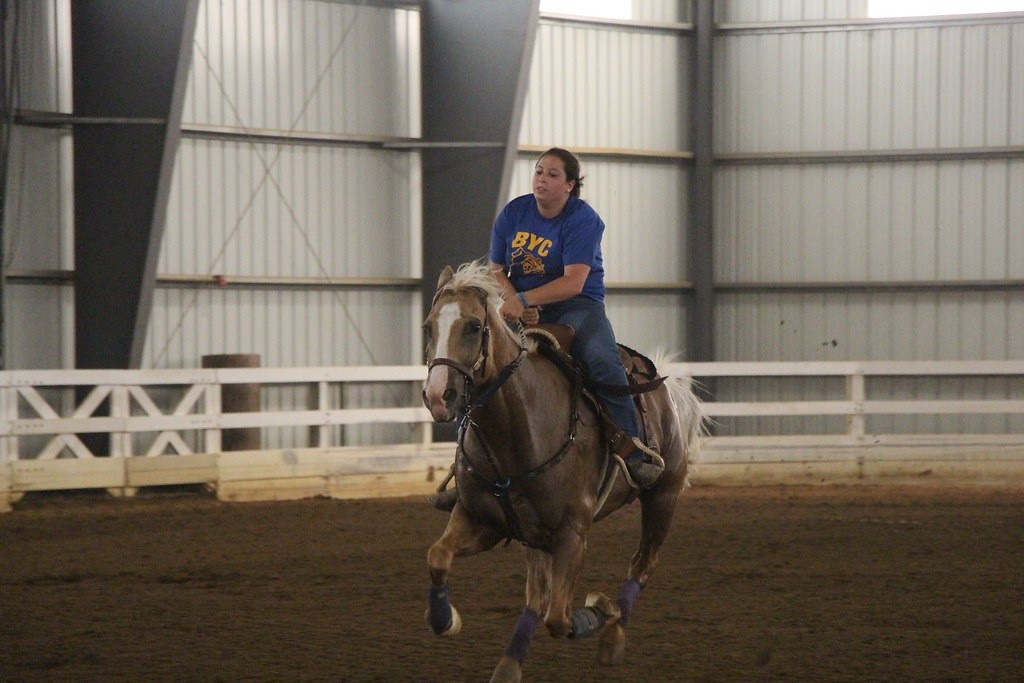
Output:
[429,486,460,511]
[632,459,658,484]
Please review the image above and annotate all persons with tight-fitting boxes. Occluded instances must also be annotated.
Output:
[426,146,666,512]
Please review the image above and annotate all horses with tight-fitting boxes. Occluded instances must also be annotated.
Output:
[418,253,729,683]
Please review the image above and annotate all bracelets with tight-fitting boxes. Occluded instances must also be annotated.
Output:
[516,291,529,309]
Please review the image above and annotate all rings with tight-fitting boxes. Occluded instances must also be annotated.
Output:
[502,316,508,320]
